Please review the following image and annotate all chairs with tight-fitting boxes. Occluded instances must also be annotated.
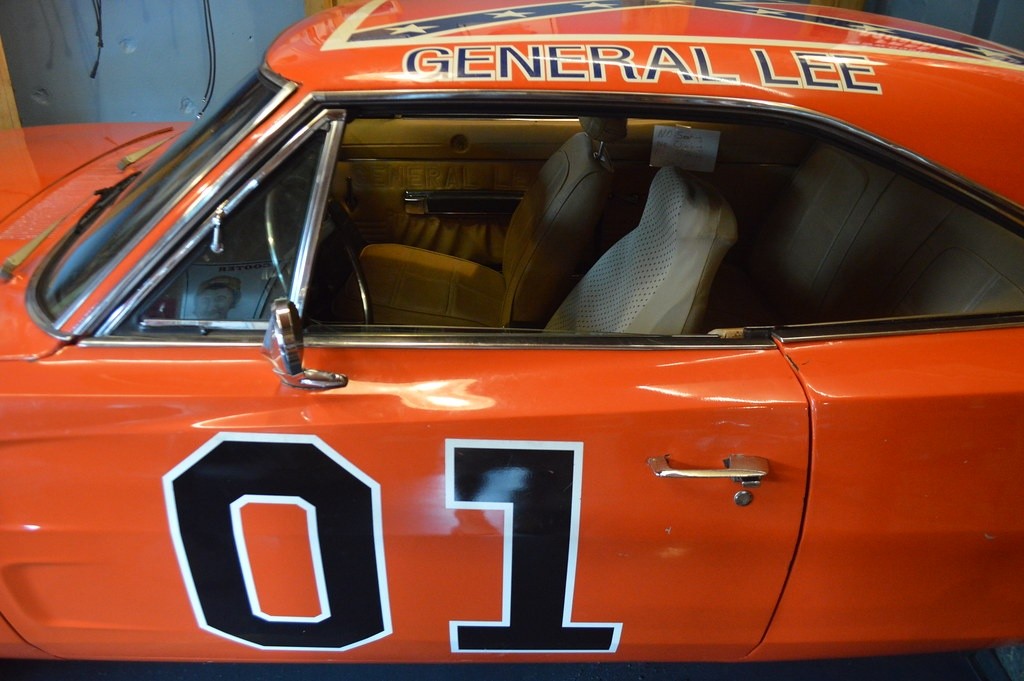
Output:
[351,116,628,334]
[542,164,738,334]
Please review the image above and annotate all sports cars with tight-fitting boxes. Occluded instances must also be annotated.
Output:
[0,1,1024,661]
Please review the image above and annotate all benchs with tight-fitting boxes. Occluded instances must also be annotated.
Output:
[693,140,1024,336]
